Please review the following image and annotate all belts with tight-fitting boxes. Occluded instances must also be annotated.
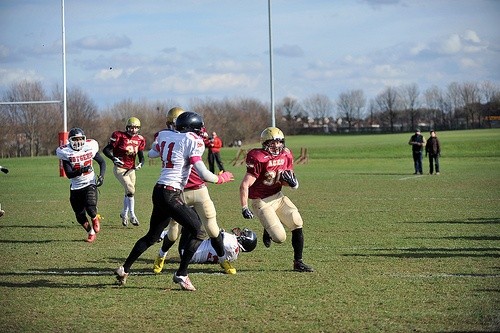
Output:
[157,183,181,193]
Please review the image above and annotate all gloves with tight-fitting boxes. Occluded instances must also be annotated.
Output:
[134,163,142,171]
[96,175,104,187]
[241,208,254,219]
[282,171,297,187]
[114,157,124,167]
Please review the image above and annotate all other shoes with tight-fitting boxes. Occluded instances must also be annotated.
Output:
[263,228,271,247]
[130,217,139,226]
[293,260,313,272]
[92,216,100,232]
[173,272,197,292]
[114,266,129,285]
[87,234,96,242]
[119,213,129,226]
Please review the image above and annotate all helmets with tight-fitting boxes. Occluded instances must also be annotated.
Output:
[167,107,185,123]
[176,111,205,133]
[126,117,141,127]
[237,228,257,252]
[68,128,86,141]
[260,126,285,144]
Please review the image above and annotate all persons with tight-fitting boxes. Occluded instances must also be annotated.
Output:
[425,130,441,175]
[55,126,105,243]
[152,107,237,276]
[205,130,227,179]
[115,112,235,293]
[240,126,314,273]
[155,225,257,264]
[102,116,146,228]
[408,128,427,175]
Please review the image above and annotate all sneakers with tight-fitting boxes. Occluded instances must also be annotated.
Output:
[221,259,236,275]
[152,254,165,274]
